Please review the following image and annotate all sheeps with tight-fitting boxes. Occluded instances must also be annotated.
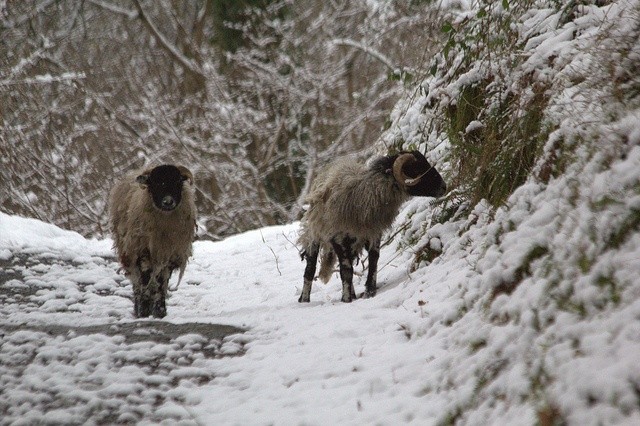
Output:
[294,149,447,303]
[107,164,198,319]
